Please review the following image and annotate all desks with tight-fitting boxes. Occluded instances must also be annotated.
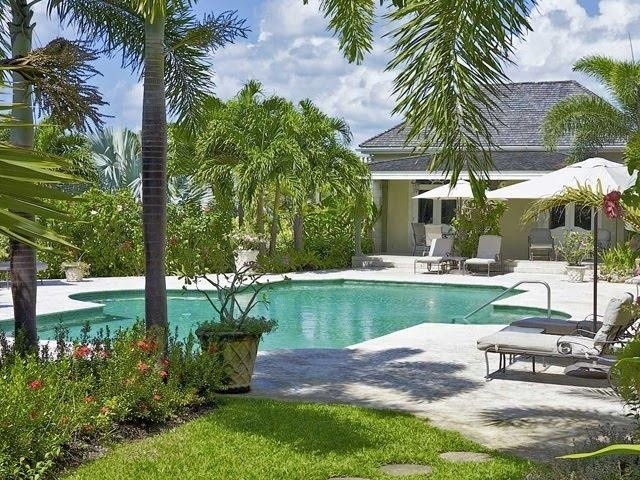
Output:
[0,261,48,289]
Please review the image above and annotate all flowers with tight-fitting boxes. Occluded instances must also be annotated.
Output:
[227,232,267,250]
[63,244,91,276]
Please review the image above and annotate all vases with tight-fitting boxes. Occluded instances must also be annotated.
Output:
[63,264,84,282]
[232,250,259,276]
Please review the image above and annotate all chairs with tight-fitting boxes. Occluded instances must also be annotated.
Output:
[411,220,640,381]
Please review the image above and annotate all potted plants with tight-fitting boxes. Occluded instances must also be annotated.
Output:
[181,260,290,394]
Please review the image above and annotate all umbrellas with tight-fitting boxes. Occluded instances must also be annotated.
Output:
[482,157,640,336]
[409,178,509,216]
